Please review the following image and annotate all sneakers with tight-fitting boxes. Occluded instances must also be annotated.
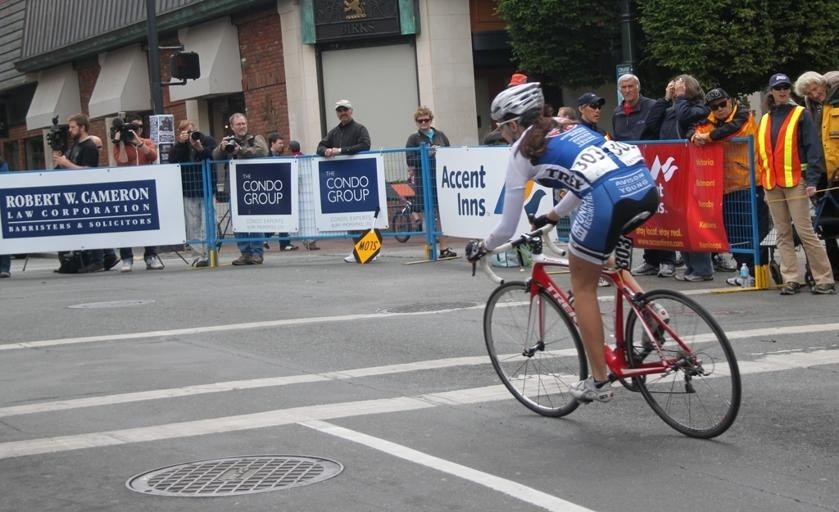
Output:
[810,282,834,294]
[780,282,799,295]
[344,253,375,263]
[630,257,714,281]
[232,253,262,266]
[642,302,670,345]
[569,377,613,402]
[78,254,133,272]
[147,258,163,270]
[726,276,755,288]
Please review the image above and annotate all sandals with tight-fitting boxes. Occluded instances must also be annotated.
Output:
[438,247,456,259]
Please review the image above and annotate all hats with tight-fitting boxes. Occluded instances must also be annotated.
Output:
[703,88,727,105]
[576,92,605,106]
[508,74,527,86]
[335,99,352,109]
[769,72,790,86]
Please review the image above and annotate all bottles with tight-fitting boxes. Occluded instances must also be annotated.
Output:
[741,261,751,289]
[567,288,576,310]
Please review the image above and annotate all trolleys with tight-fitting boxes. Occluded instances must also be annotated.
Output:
[762,169,839,287]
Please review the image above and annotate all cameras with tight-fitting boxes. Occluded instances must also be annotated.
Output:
[222,123,243,153]
[186,130,202,143]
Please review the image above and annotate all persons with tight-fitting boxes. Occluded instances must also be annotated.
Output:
[317,99,382,259]
[0,158,11,278]
[615,74,839,295]
[405,107,458,257]
[168,119,219,268]
[53,114,125,270]
[544,92,606,137]
[264,134,320,250]
[484,128,510,147]
[111,113,165,270]
[212,111,270,264]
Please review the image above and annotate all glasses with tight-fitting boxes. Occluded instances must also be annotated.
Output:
[709,101,727,111]
[589,103,601,110]
[337,107,347,112]
[416,118,430,123]
[773,84,789,90]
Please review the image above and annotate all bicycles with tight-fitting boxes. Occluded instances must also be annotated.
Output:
[462,212,709,439]
[392,196,440,242]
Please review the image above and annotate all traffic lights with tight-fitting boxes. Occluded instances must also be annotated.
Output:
[169,51,200,80]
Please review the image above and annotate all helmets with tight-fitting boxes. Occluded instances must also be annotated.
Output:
[491,82,544,122]
